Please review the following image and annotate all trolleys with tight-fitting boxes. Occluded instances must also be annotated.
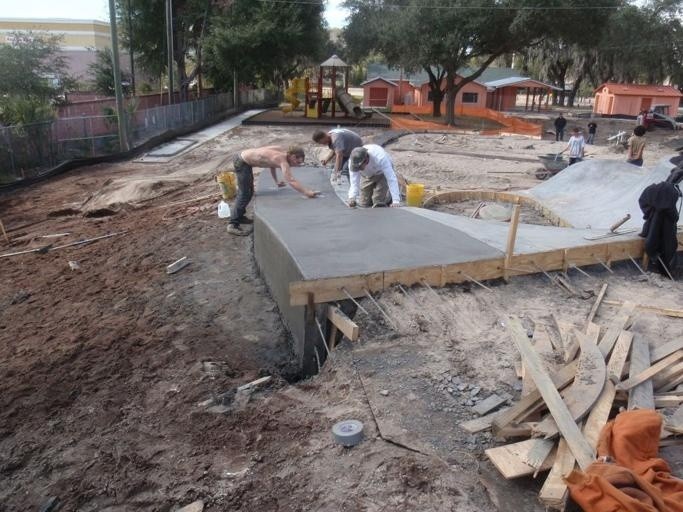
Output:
[535,153,569,180]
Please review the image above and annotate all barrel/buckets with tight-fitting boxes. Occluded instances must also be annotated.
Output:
[216,171,237,200]
[406,183,423,204]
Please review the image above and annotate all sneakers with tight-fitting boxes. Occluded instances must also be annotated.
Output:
[226,223,250,236]
[240,215,254,224]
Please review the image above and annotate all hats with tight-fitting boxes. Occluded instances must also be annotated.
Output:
[349,146,368,172]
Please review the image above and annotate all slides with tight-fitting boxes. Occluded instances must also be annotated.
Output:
[282,87,305,113]
[335,87,365,118]
[307,98,318,117]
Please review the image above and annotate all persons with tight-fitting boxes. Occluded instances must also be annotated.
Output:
[310,127,363,176]
[226,145,321,237]
[585,120,597,145]
[625,125,647,167]
[554,110,566,142]
[344,143,401,208]
[559,128,585,166]
[636,111,647,131]
